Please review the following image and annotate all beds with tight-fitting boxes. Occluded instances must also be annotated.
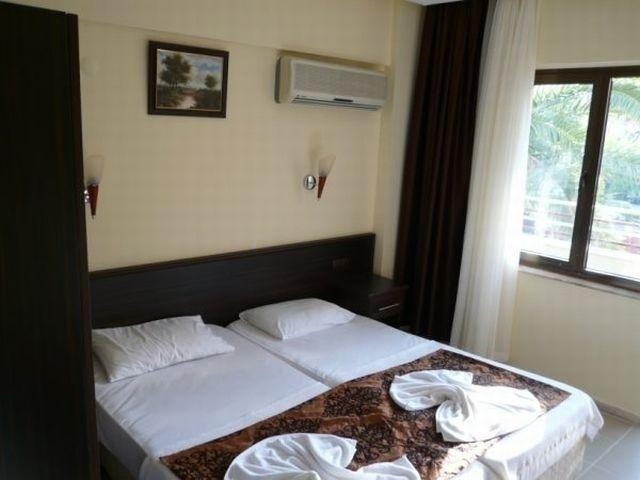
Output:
[88,232,605,480]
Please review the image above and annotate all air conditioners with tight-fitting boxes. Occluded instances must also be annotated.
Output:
[274,55,387,111]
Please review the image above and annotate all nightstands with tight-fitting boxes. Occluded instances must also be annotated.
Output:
[362,274,404,332]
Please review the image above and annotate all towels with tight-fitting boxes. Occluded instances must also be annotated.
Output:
[224,432,422,480]
[387,369,542,443]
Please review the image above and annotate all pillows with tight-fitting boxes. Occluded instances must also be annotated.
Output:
[91,314,235,383]
[239,298,355,340]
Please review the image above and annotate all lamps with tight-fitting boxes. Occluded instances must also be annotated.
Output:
[82,153,104,221]
[303,153,337,206]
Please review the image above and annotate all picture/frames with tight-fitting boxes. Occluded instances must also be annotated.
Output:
[148,40,229,119]
